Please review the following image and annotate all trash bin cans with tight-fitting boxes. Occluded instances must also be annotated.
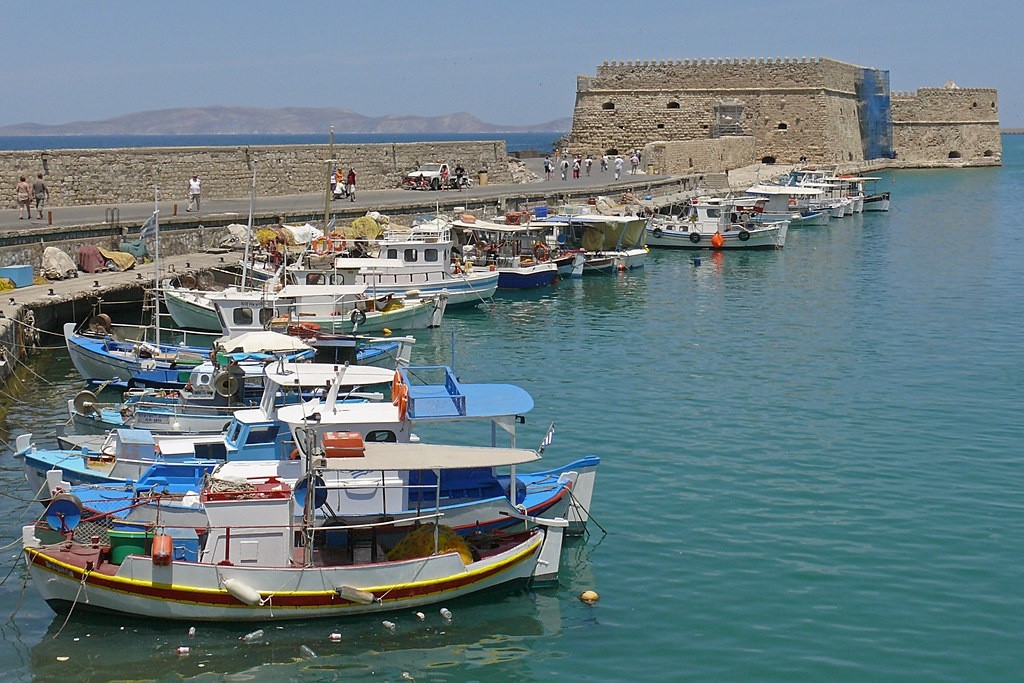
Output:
[647,163,653,175]
[479,170,487,185]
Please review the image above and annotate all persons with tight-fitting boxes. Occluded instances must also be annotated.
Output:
[560,155,569,181]
[440,165,449,192]
[572,156,582,181]
[614,155,624,181]
[585,155,593,176]
[186,174,201,213]
[455,163,465,192]
[630,152,639,175]
[543,156,555,183]
[636,149,641,162]
[31,173,50,220]
[554,149,560,163]
[600,153,610,172]
[346,166,356,202]
[16,175,34,219]
[330,170,337,201]
[335,168,346,192]
[562,149,569,160]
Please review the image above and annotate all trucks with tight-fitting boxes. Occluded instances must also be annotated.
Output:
[408,163,467,189]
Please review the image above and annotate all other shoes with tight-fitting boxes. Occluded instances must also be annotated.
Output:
[186,209,190,212]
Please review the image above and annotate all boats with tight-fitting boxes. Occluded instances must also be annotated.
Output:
[643,196,792,249]
[746,165,892,226]
[14,184,648,538]
[24,427,567,622]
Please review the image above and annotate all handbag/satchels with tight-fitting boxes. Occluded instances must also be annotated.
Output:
[589,160,592,166]
[575,168,578,171]
[549,163,552,171]
[565,162,568,168]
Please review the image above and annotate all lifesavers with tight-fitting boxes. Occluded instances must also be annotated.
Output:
[738,231,750,241]
[399,384,408,421]
[329,234,346,250]
[789,200,796,205]
[506,213,520,225]
[690,232,700,243]
[222,421,232,432]
[351,310,366,325]
[739,212,750,222]
[313,236,332,256]
[392,370,402,406]
[534,244,548,262]
[653,228,663,238]
[290,449,299,460]
[183,271,215,290]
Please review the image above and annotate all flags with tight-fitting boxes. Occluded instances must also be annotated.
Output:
[541,426,555,448]
[326,218,335,233]
[140,212,156,240]
